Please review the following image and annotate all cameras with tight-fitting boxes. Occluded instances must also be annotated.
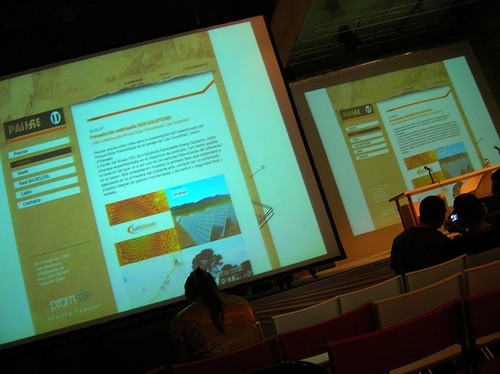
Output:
[448,212,459,222]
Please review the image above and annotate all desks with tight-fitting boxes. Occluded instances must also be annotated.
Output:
[389,164,500,233]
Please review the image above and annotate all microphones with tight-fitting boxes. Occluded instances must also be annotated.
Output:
[494,145,499,150]
[424,166,430,170]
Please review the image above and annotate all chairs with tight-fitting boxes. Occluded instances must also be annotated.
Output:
[146,248,500,374]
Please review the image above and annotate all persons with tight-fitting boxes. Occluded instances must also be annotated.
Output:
[170,267,262,360]
[390,195,457,274]
[445,194,500,254]
